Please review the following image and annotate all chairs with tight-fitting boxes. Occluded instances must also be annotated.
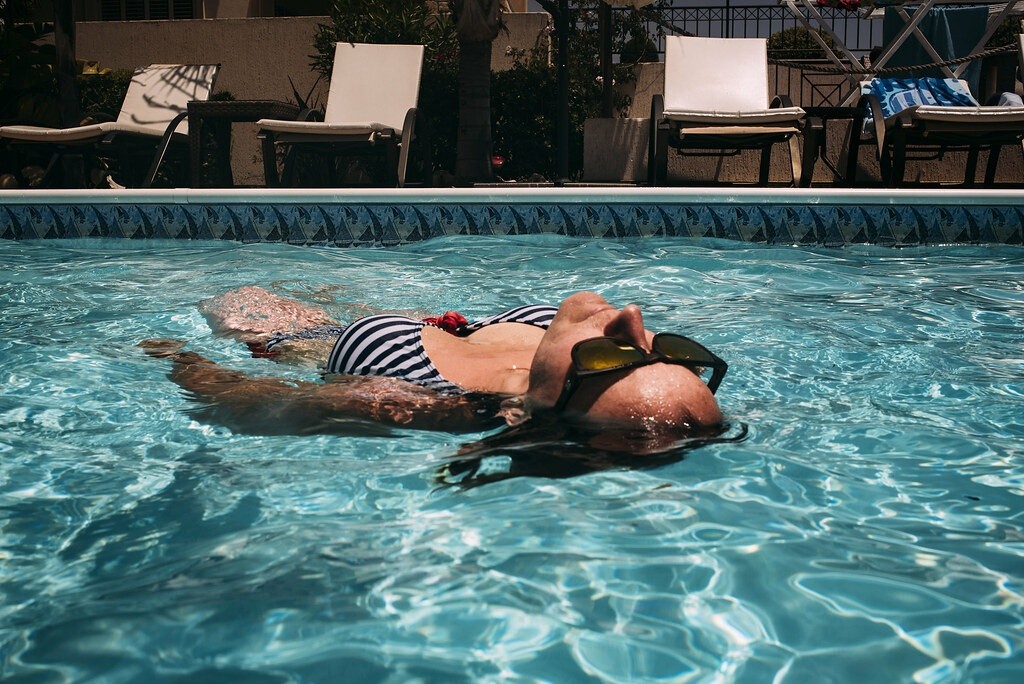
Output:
[0,63,222,189]
[846,79,1024,187]
[255,42,429,188]
[649,35,823,188]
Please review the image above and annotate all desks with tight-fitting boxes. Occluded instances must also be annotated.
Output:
[187,100,300,188]
[800,107,870,187]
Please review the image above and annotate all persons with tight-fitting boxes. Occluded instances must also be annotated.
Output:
[327,292,721,433]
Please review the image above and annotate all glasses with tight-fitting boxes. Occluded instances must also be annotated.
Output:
[550,332,728,428]
